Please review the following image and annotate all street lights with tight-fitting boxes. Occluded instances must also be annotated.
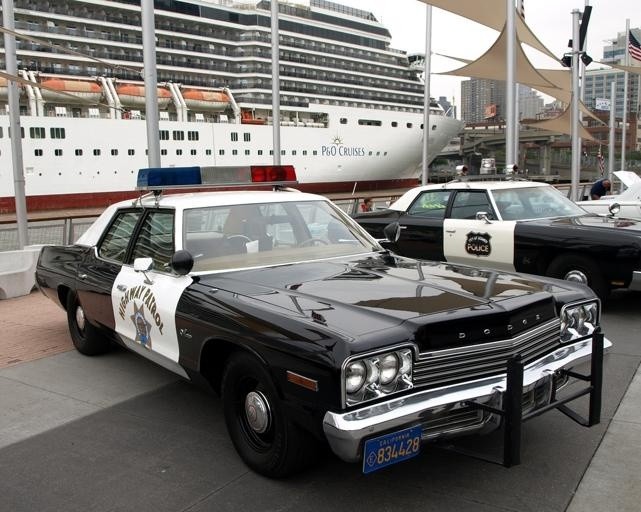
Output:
[563,49,591,204]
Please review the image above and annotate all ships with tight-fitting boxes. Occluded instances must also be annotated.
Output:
[0,1,469,216]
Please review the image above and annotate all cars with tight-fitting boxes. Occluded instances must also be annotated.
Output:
[571,169,641,231]
[344,181,641,303]
[36,163,613,478]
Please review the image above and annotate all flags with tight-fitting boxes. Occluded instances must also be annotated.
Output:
[629,31,641,70]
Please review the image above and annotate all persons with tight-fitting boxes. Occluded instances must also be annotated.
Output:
[590,179,612,200]
[362,198,374,212]
